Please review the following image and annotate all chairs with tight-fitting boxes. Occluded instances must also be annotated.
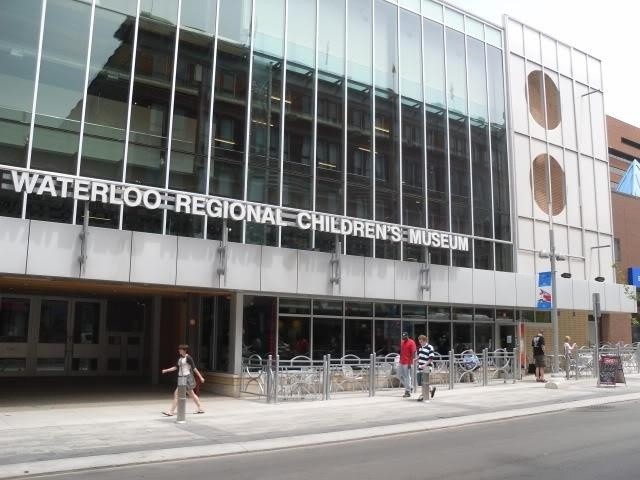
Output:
[246,349,509,399]
[549,341,639,376]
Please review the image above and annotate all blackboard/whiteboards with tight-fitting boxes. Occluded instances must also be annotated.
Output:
[597,355,619,385]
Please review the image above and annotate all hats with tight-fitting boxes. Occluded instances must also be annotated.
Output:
[401,332,408,338]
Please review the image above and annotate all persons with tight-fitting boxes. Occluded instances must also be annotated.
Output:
[295,336,309,353]
[396,332,418,398]
[562,335,578,381]
[161,343,207,416]
[460,343,481,383]
[413,334,437,402]
[250,336,263,353]
[531,330,549,383]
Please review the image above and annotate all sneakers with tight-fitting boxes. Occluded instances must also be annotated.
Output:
[429,386,437,398]
[536,378,548,382]
[417,395,424,401]
[403,387,413,397]
[194,409,204,413]
[161,410,173,417]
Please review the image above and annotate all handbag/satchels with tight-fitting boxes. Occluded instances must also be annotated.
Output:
[185,374,197,389]
